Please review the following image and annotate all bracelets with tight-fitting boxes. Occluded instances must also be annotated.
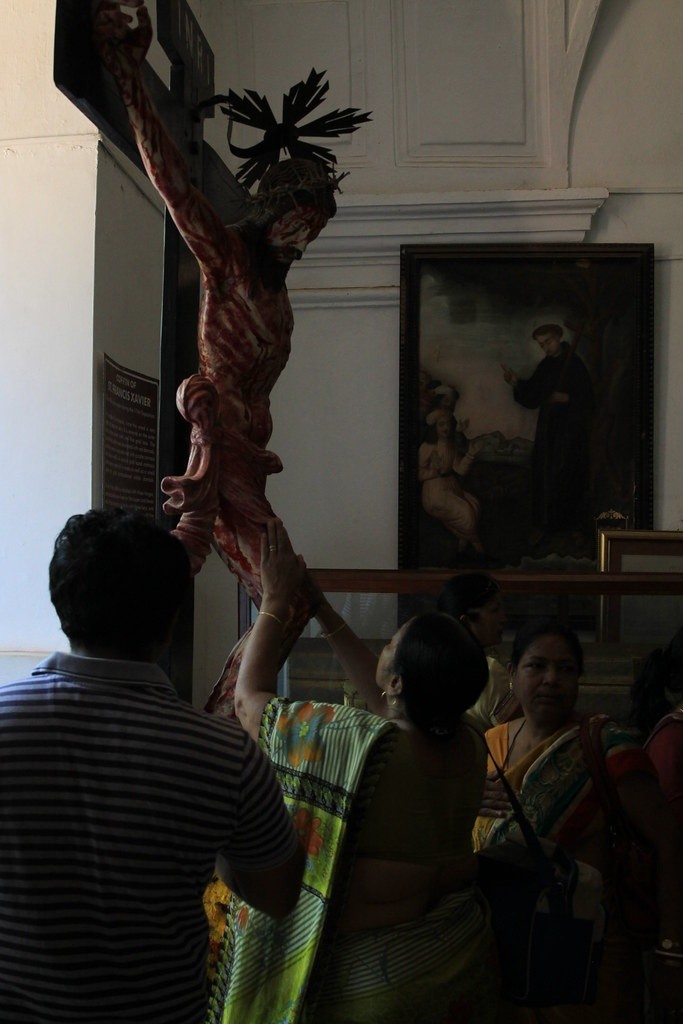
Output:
[320,622,347,639]
[655,956,683,968]
[259,611,284,626]
[654,950,683,958]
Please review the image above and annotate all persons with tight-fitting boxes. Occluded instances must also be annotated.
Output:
[198,517,502,1024]
[0,506,309,1024]
[436,567,683,1024]
[94,0,340,721]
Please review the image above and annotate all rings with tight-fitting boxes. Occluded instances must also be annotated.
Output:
[269,545,277,552]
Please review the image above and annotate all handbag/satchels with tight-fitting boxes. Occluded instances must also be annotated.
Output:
[472,845,605,1009]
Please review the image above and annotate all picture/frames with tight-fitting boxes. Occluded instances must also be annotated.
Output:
[396,242,656,645]
[596,527,683,645]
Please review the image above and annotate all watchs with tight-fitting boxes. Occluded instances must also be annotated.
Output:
[652,934,682,948]
